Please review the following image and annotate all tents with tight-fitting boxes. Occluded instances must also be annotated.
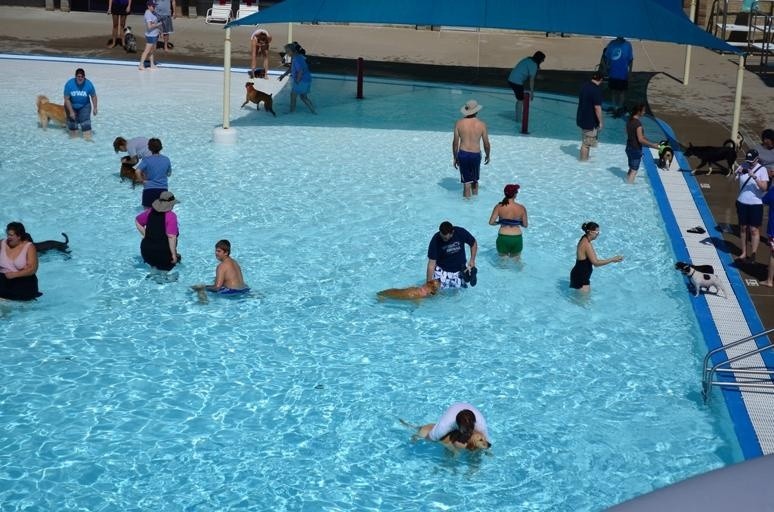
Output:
[223,0,746,56]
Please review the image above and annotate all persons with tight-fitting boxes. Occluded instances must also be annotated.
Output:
[489,184,528,261]
[427,222,477,291]
[760,175,774,286]
[134,193,181,269]
[576,71,602,162]
[64,69,96,141]
[278,44,319,116]
[113,136,150,165]
[756,130,774,174]
[603,35,633,119]
[251,29,272,79]
[138,1,162,71]
[107,0,130,49]
[0,222,37,301]
[507,51,546,121]
[428,405,488,446]
[452,100,490,199]
[135,139,171,211]
[735,150,768,261]
[570,222,624,295]
[625,103,660,181]
[205,241,245,295]
[153,0,176,51]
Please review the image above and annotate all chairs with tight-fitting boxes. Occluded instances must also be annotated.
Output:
[205,1,232,26]
[236,0,259,28]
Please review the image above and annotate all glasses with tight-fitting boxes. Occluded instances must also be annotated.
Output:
[591,231,600,234]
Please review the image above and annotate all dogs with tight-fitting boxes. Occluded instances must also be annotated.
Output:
[121,156,144,188]
[33,233,74,264]
[124,25,138,53]
[377,278,444,306]
[658,137,676,170]
[242,81,278,118]
[681,264,728,298]
[37,94,68,131]
[684,138,738,178]
[191,284,210,305]
[398,416,492,461]
[737,130,745,151]
[676,261,714,293]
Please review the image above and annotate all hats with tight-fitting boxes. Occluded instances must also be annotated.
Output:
[152,191,181,213]
[744,148,760,164]
[146,1,160,6]
[460,99,483,117]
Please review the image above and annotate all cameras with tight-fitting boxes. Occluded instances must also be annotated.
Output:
[742,164,748,174]
